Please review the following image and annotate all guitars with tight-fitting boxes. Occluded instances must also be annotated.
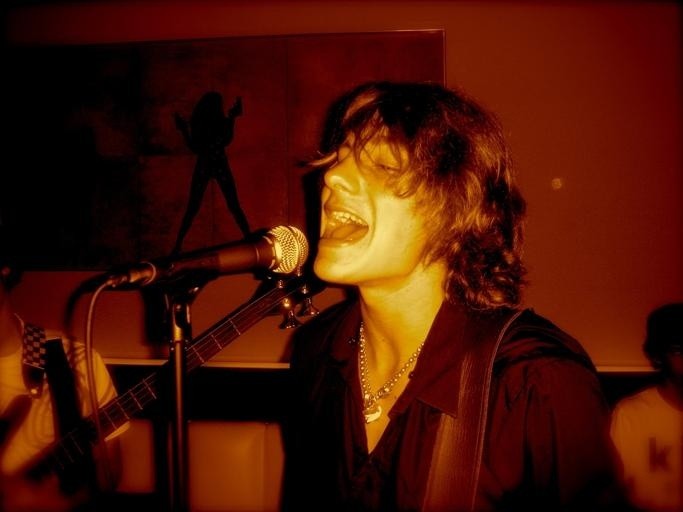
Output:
[2,267,323,509]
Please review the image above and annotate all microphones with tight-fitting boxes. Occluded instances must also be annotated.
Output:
[128,225,310,288]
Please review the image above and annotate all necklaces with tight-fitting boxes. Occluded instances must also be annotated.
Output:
[358,321,424,424]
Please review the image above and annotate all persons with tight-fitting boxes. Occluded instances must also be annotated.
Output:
[611,304,683,512]
[168,91,250,258]
[0,253,133,492]
[274,79,633,512]
